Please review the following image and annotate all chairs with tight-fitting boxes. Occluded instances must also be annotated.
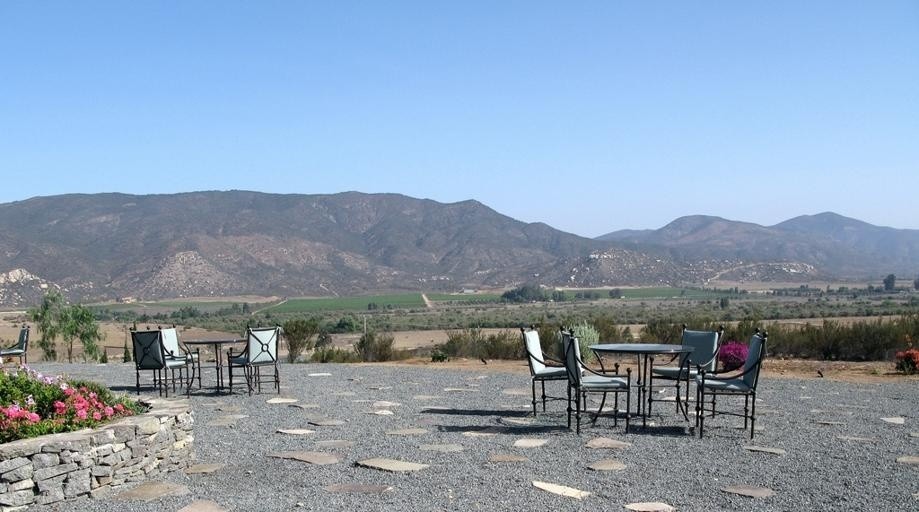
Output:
[226,322,282,395]
[0,325,30,365]
[520,323,633,434]
[129,322,202,398]
[648,322,769,439]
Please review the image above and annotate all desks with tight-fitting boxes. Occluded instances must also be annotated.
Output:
[586,342,697,423]
[182,336,255,392]
